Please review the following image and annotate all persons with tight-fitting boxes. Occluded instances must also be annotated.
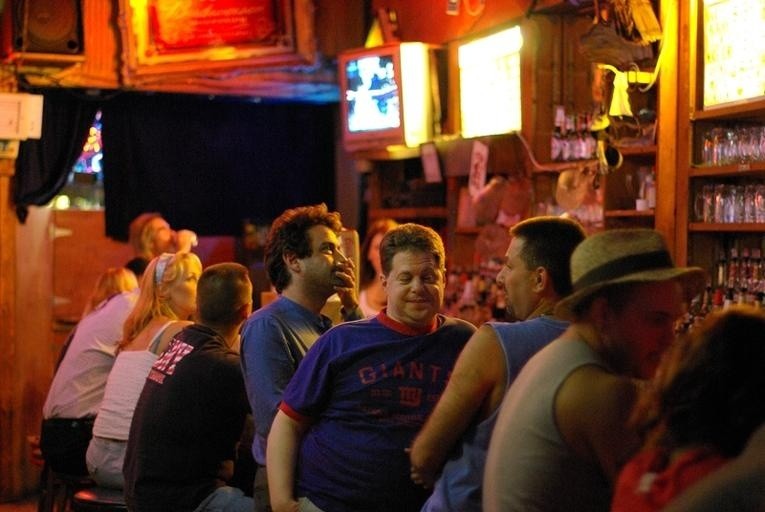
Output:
[85,250,205,488]
[265,223,480,512]
[38,265,141,477]
[611,300,765,511]
[79,207,277,318]
[401,214,593,511]
[482,227,710,512]
[238,203,367,511]
[361,218,401,320]
[122,260,258,511]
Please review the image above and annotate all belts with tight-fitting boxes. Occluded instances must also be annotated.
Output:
[42,418,91,427]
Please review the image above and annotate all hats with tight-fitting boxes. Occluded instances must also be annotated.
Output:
[553,228,705,319]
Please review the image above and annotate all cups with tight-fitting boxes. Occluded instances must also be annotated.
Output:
[635,198,651,213]
[700,122,765,167]
[694,183,765,224]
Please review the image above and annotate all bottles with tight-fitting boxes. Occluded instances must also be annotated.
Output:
[647,168,656,210]
[548,103,595,160]
[698,248,765,316]
[444,260,507,322]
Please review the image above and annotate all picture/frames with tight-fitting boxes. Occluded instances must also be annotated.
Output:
[110,0,318,87]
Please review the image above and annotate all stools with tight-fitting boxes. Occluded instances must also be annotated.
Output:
[26,434,128,512]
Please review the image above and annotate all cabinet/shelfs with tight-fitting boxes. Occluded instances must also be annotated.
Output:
[534,141,660,220]
[685,98,764,306]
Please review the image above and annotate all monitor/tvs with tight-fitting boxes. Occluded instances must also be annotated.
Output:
[338,41,431,152]
